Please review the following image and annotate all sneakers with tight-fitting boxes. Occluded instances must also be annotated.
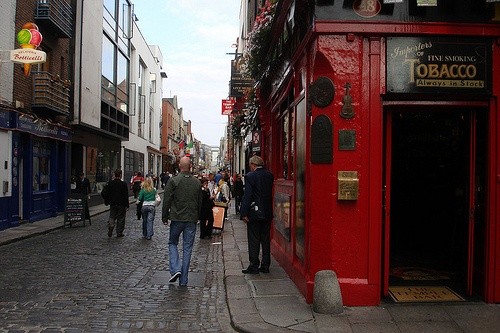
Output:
[181,284,186,286]
[169,272,182,282]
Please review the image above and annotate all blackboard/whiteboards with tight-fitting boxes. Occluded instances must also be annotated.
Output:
[64,193,85,221]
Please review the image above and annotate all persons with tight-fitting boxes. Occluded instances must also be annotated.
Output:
[162,156,202,287]
[71,168,243,240]
[242,156,273,274]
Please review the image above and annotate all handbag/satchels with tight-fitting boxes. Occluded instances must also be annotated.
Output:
[154,190,161,207]
[221,196,230,203]
[240,199,265,220]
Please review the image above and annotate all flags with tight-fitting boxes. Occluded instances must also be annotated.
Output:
[178,139,192,151]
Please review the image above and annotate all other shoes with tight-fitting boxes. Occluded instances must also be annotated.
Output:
[225,218,229,221]
[258,267,264,272]
[242,269,258,274]
[145,236,151,240]
[117,233,124,237]
[108,226,112,237]
